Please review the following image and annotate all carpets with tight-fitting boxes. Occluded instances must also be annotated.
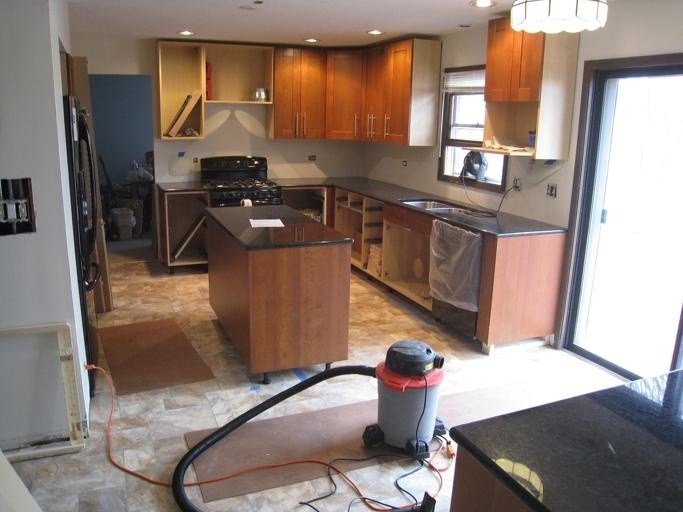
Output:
[180,399,446,506]
[97,318,217,396]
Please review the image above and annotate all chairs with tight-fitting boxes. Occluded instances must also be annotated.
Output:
[99,156,145,237]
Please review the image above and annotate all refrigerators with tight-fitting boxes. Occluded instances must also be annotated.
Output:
[63,93,102,399]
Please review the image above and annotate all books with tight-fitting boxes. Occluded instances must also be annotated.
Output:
[163,89,200,138]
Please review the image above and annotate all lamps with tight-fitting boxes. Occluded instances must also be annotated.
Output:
[510,0,609,35]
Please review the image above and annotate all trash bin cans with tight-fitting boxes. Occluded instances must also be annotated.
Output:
[112,208,136,241]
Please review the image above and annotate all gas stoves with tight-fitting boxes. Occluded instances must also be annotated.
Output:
[203,176,282,204]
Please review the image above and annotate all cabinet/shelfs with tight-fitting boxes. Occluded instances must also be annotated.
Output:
[385,218,434,308]
[361,197,382,278]
[326,44,364,142]
[154,36,274,143]
[335,188,362,263]
[156,178,332,273]
[429,222,483,340]
[276,43,325,140]
[365,38,441,148]
[483,13,544,105]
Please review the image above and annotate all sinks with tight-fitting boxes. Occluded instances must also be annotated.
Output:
[398,197,456,210]
[425,208,474,215]
